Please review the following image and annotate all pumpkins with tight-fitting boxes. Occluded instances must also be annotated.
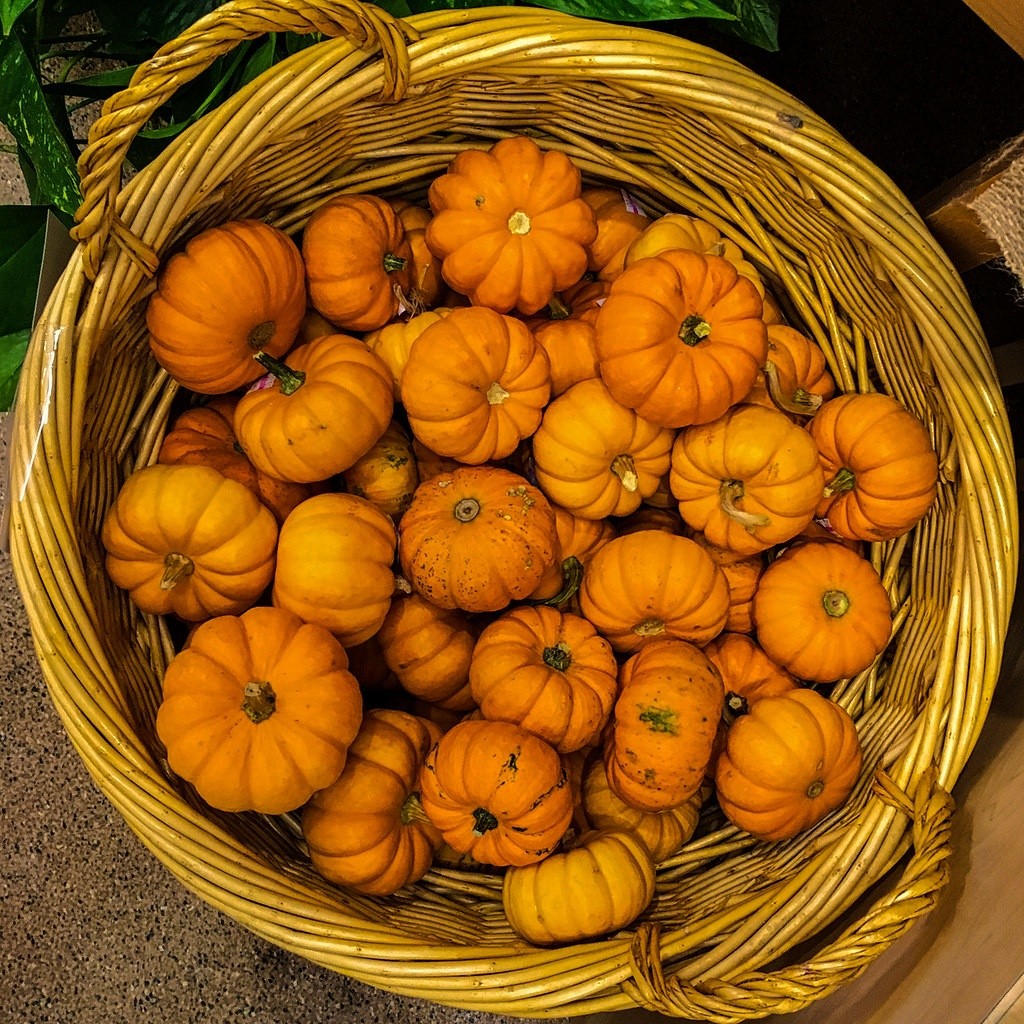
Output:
[99,134,939,946]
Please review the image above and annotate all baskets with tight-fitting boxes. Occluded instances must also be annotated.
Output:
[7,0,1019,1024]
